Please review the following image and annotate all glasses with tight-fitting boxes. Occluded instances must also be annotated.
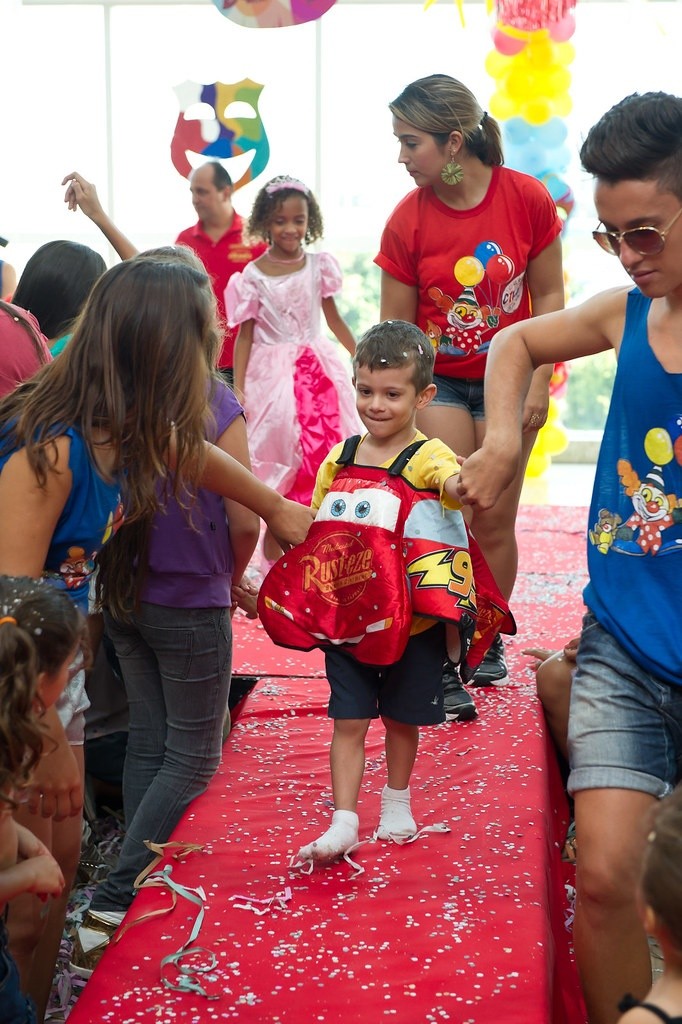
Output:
[592,206,682,256]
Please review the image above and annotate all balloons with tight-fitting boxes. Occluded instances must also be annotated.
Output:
[479,0,581,479]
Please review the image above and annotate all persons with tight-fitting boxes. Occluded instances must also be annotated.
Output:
[1,259,18,302]
[457,90,680,1022]
[371,74,566,682]
[222,175,358,569]
[1,299,54,408]
[1,255,220,1022]
[615,788,681,1023]
[174,162,272,392]
[81,248,270,930]
[8,238,107,360]
[254,321,519,861]
[520,632,584,861]
[0,574,82,1021]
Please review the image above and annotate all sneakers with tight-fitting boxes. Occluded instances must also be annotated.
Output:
[467,631,511,686]
[439,660,479,722]
[87,901,127,928]
[68,912,127,980]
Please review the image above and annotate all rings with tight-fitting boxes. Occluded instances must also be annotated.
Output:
[529,413,539,426]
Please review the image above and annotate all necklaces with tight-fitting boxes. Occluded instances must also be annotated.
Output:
[266,245,306,265]
